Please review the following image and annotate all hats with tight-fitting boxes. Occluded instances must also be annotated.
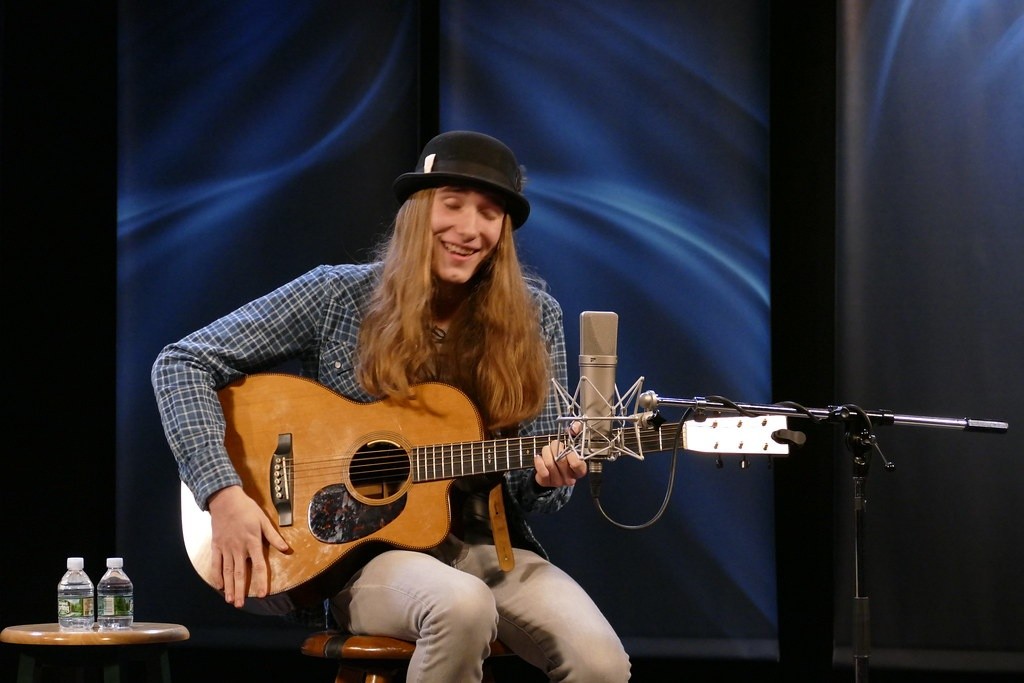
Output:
[393,130,532,231]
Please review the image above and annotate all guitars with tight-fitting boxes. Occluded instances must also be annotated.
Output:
[180,373,807,617]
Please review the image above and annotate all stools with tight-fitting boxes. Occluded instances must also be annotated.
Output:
[0,623,190,683]
[301,634,518,683]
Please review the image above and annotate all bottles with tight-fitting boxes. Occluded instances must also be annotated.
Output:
[57,557,95,631]
[97,558,134,629]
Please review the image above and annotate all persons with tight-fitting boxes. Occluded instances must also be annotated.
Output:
[150,130,632,683]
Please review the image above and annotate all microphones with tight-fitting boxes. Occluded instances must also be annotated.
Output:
[579,311,618,498]
